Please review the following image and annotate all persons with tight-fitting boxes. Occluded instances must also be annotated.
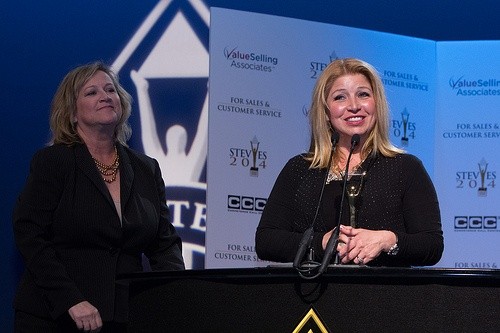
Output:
[14,60,186,333]
[254,58,444,268]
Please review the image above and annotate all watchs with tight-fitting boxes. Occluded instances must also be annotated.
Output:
[386,234,399,255]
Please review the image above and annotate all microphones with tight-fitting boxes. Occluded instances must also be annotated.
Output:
[319,134,361,274]
[293,134,339,269]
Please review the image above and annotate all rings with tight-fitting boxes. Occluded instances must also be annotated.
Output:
[357,257,362,262]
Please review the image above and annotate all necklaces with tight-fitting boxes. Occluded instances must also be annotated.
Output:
[92,145,119,184]
